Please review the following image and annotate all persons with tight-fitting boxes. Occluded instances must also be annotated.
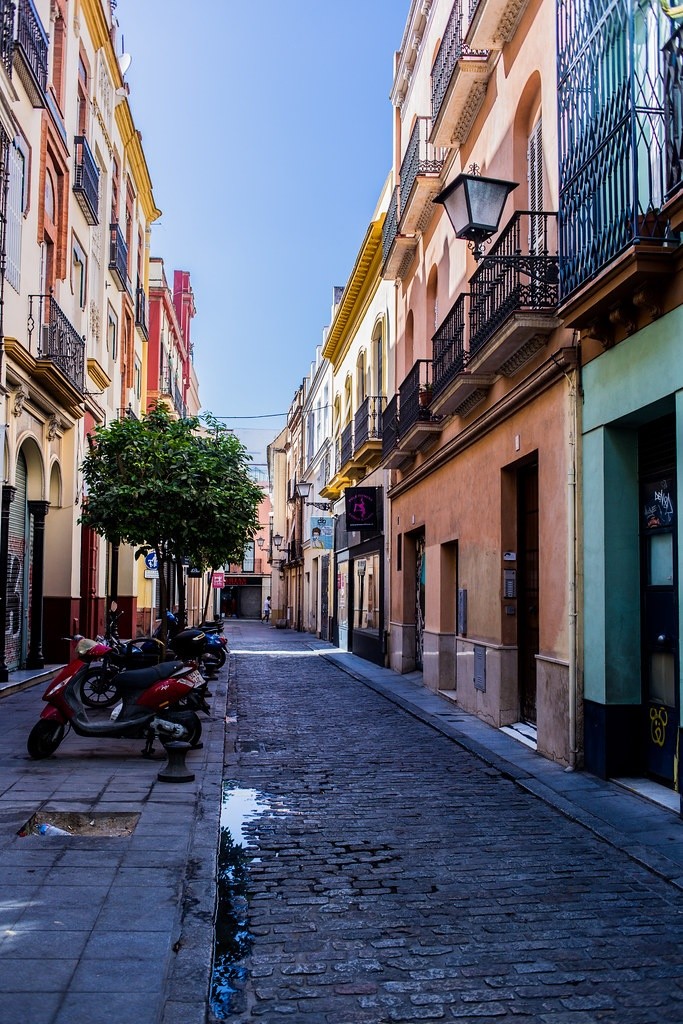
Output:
[261,596,272,625]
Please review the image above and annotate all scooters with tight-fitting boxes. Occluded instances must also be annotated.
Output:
[27,601,230,759]
